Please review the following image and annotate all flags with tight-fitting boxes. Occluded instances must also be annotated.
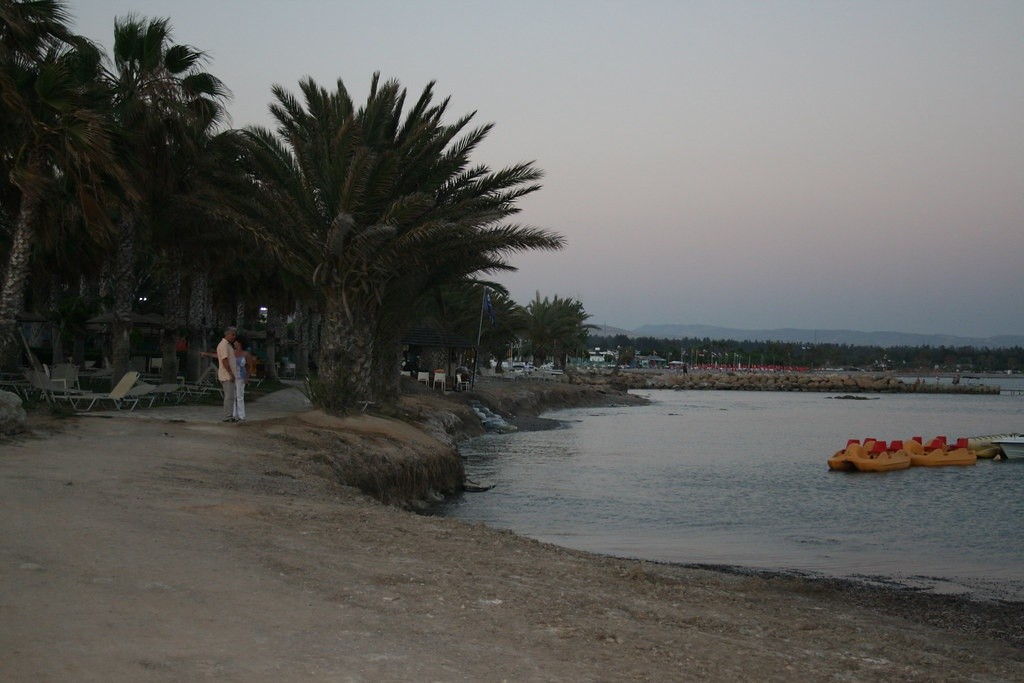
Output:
[484,288,496,327]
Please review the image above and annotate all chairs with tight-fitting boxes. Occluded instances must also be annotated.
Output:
[400,371,469,391]
[845,435,968,455]
[479,367,561,384]
[0,357,259,411]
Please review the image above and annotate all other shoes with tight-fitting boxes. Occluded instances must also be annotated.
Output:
[238,417,246,421]
[223,418,239,422]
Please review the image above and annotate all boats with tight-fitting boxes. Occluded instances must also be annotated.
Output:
[903,433,979,466]
[826,436,912,473]
[990,432,1024,460]
[967,431,1022,459]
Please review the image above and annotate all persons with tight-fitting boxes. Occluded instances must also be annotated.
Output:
[217,326,253,421]
[681,362,687,376]
